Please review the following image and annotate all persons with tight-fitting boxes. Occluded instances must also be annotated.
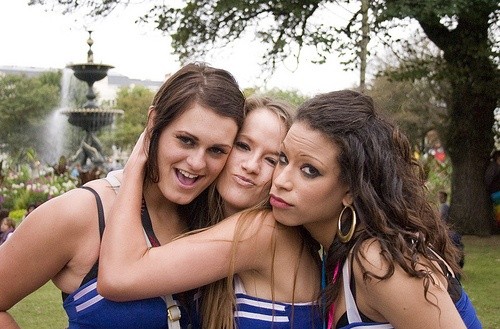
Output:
[414,143,500,264]
[268,89,500,329]
[97,95,324,329]
[0,63,246,329]
